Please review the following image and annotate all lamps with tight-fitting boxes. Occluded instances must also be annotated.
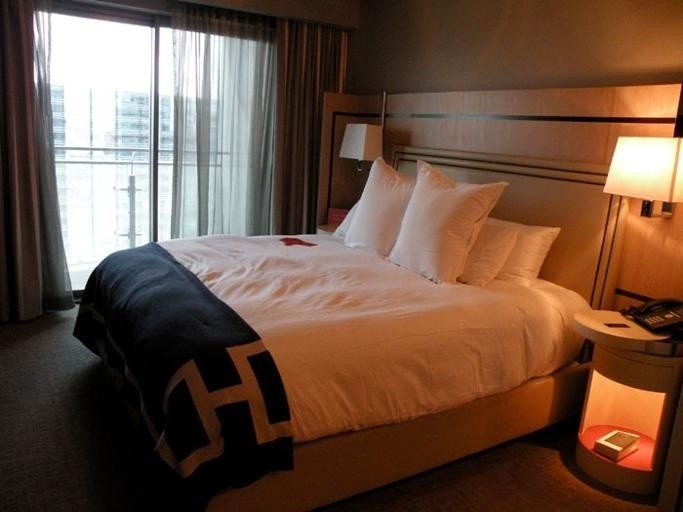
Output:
[337,90,386,173]
[601,83,682,223]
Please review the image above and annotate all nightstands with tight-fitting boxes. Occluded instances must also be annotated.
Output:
[316,222,340,239]
[563,307,682,511]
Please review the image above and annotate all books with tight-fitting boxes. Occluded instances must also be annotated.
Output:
[593,428,641,464]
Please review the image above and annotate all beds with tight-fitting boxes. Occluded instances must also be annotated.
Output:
[67,140,638,512]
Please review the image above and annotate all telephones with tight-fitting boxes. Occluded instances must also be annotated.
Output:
[633,298,683,332]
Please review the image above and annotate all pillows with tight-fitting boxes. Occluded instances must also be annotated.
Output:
[342,156,416,257]
[330,200,361,239]
[385,158,509,288]
[452,215,521,289]
[495,222,563,290]
